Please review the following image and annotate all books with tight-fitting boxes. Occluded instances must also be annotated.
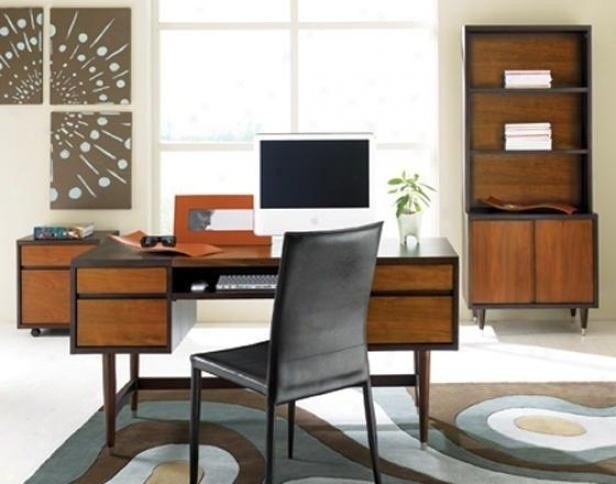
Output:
[33,224,94,241]
[503,70,553,89]
[503,123,552,151]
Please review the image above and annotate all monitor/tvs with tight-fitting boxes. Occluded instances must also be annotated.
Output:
[254,132,376,236]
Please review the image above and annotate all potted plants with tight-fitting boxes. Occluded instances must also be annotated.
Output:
[388,171,438,247]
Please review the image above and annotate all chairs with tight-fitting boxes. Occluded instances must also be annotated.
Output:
[189,221,385,484]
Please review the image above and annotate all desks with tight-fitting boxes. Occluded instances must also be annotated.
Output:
[70,237,458,454]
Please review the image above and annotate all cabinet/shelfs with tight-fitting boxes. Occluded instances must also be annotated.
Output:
[462,24,598,332]
[16,231,120,336]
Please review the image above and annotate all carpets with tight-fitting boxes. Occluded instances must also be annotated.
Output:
[24,377,616,484]
